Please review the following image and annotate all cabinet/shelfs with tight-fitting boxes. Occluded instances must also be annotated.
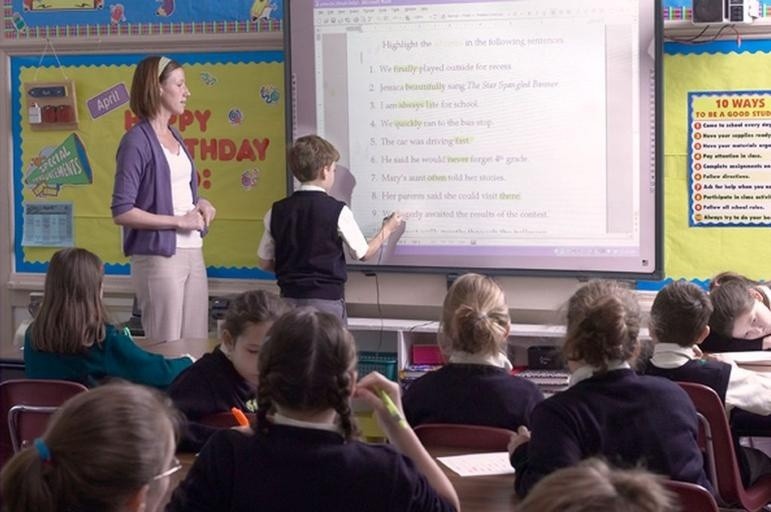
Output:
[345,319,651,396]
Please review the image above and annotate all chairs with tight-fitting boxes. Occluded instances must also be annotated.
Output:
[0,378,92,468]
[203,411,259,430]
[675,381,771,512]
[111,294,144,336]
[662,481,719,512]
[411,424,512,458]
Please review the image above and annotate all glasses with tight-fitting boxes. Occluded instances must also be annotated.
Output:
[123,455,182,502]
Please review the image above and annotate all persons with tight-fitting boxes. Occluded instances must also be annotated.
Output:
[256,135,407,327]
[707,272,771,351]
[23,247,196,390]
[512,458,681,512]
[637,281,771,490]
[163,306,460,512]
[507,279,723,506]
[0,383,192,512]
[110,56,216,340]
[166,290,293,453]
[398,273,544,432]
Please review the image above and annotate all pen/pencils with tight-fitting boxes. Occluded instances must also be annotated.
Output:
[123,326,133,340]
[231,406,250,427]
[371,381,411,432]
